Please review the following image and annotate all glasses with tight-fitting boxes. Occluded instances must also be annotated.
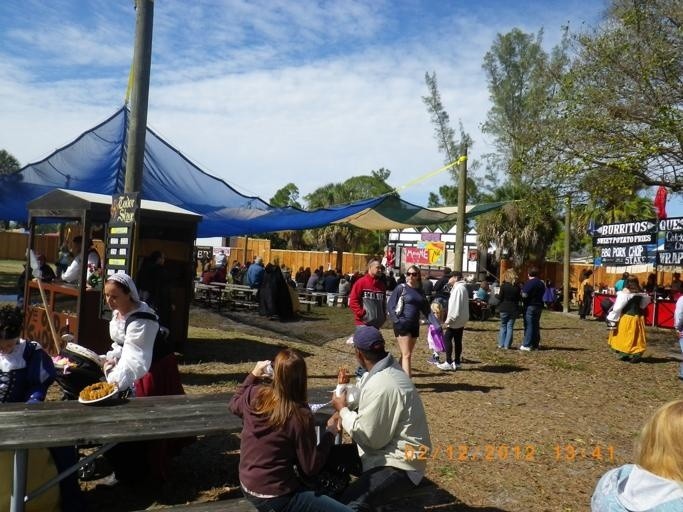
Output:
[406,271,418,276]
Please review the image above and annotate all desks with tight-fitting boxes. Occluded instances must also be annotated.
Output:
[0,386,341,511]
[591,288,676,328]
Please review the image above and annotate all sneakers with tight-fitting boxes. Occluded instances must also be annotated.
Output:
[520,345,531,351]
[427,354,461,370]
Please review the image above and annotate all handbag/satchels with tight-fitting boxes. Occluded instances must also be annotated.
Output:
[386,295,403,315]
[311,463,352,496]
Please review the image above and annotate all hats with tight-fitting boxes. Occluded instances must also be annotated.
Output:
[346,325,385,353]
[444,271,462,277]
[107,272,139,302]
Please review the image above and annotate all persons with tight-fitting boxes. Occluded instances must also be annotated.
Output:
[439,270,471,370]
[331,325,432,511]
[101,271,215,500]
[427,302,444,364]
[1,303,58,511]
[673,294,682,379]
[135,250,179,356]
[205,245,360,310]
[492,266,522,351]
[346,257,390,377]
[576,267,682,320]
[604,273,655,364]
[588,396,682,511]
[518,266,548,352]
[226,348,356,511]
[386,263,444,381]
[17,251,56,301]
[59,235,100,284]
[381,249,571,315]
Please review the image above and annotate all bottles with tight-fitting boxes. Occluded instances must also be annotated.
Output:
[256,361,273,375]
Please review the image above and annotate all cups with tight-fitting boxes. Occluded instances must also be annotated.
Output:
[609,288,614,294]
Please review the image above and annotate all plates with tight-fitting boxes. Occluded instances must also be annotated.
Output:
[78,385,119,405]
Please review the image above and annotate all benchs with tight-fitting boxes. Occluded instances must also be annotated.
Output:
[134,477,474,511]
[193,282,350,314]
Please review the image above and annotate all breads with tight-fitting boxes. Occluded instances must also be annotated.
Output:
[338,368,350,384]
[79,382,114,400]
